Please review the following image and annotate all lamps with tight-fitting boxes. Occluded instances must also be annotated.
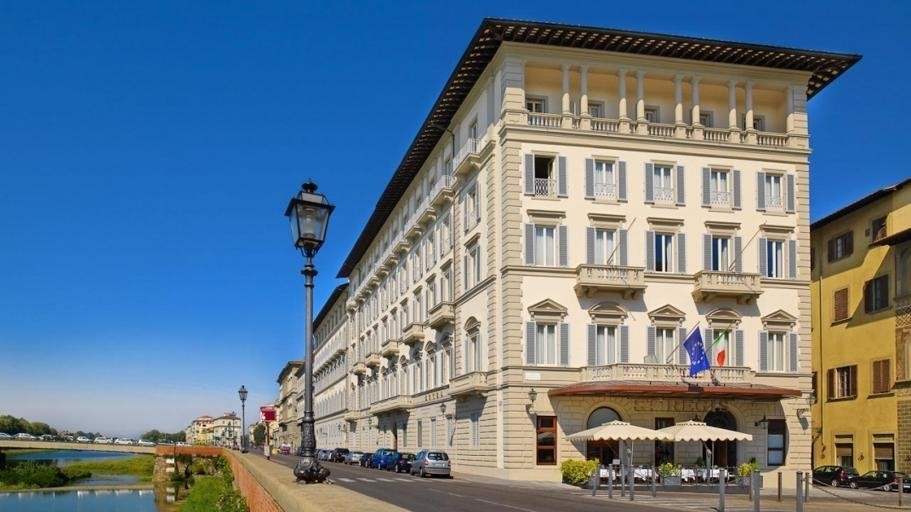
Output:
[797,408,812,419]
[337,424,341,430]
[526,389,537,413]
[440,402,446,415]
[368,417,372,429]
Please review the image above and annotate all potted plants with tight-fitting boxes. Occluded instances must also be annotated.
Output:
[658,462,682,485]
[561,458,600,488]
[739,462,751,487]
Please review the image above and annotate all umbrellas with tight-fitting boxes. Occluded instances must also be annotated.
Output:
[560,419,674,468]
[652,419,755,485]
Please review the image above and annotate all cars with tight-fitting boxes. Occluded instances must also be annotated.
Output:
[0,432,191,447]
[279,445,451,476]
[812,465,911,492]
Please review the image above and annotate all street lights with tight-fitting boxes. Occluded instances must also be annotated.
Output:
[238,385,249,453]
[229,411,237,447]
[285,180,335,479]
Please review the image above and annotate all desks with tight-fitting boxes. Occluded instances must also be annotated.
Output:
[600,469,730,484]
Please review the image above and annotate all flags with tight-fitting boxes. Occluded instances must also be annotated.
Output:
[682,324,712,379]
[711,331,731,366]
